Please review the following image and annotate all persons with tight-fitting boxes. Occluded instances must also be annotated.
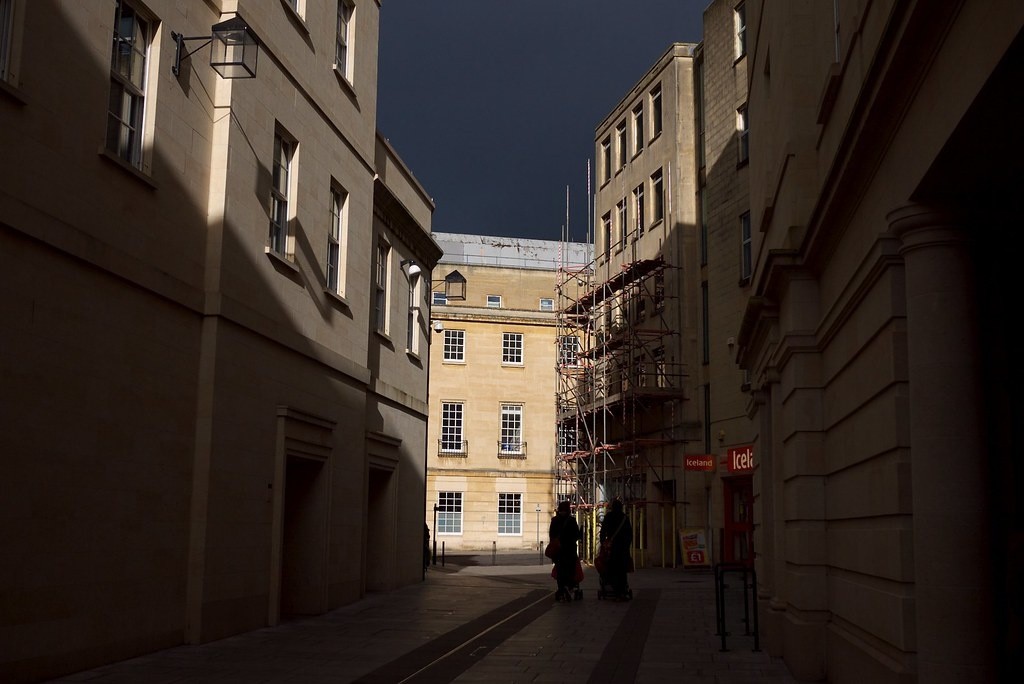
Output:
[599,498,633,601]
[550,501,580,603]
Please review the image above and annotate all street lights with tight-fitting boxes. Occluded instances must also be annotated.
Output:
[535,503,541,551]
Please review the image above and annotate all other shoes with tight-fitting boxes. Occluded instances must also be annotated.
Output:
[563,586,572,603]
[559,596,565,603]
[623,594,630,601]
[614,597,620,603]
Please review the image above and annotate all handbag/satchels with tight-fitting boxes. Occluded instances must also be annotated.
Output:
[594,538,613,575]
[545,538,561,558]
